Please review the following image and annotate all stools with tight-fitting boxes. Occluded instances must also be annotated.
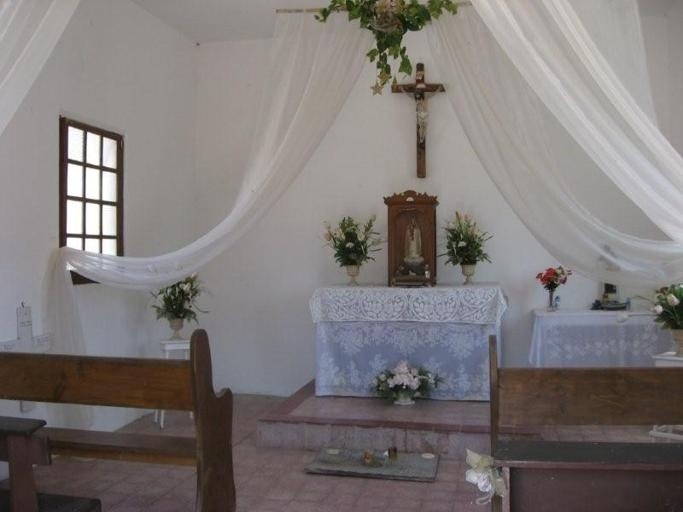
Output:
[153,339,196,427]
[649,351,683,439]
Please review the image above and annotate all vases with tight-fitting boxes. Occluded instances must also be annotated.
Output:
[346,264,360,286]
[169,318,183,338]
[545,292,555,311]
[671,329,683,356]
[459,264,477,283]
[392,391,416,405]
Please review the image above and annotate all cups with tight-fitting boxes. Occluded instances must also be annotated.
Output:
[388,447,397,458]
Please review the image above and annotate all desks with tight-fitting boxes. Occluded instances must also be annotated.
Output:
[309,285,506,400]
[530,309,675,368]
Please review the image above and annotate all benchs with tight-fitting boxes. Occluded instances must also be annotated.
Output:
[487,333,683,512]
[0,416,102,512]
[0,329,237,512]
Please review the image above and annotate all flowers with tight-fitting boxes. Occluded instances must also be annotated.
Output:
[536,265,574,291]
[153,273,209,321]
[371,360,444,397]
[436,212,497,264]
[320,214,386,263]
[650,283,683,329]
